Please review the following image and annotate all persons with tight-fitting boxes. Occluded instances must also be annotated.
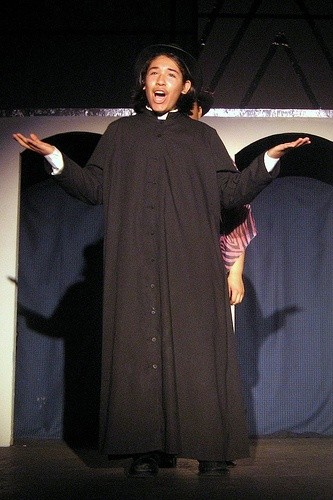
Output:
[12,44,311,477]
[152,88,258,467]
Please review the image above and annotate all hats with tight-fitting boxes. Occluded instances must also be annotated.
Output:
[199,86,214,115]
[133,44,203,97]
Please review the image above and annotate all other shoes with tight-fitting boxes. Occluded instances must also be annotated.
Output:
[129,452,160,479]
[197,460,230,477]
